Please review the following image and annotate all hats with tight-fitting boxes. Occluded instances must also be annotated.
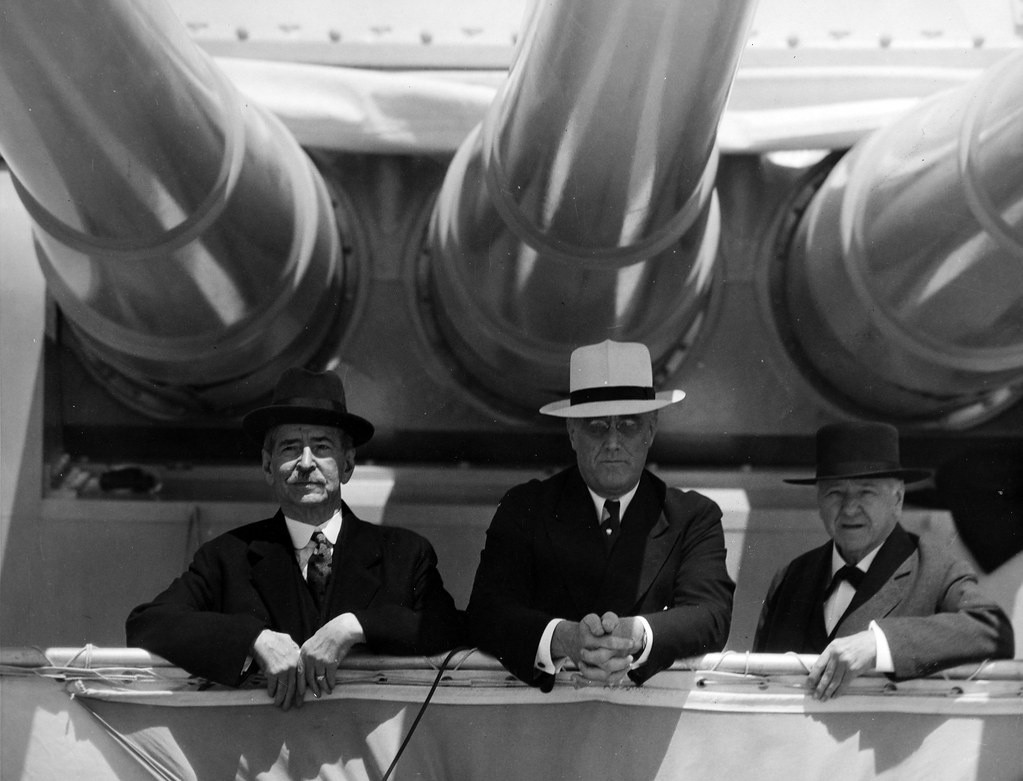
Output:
[783,421,931,485]
[538,338,685,418]
[241,367,375,448]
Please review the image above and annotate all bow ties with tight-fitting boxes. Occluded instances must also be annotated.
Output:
[833,565,866,589]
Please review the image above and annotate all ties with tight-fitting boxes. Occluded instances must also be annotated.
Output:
[601,499,618,557]
[307,532,332,613]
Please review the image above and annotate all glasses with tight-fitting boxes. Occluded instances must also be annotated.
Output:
[579,418,647,436]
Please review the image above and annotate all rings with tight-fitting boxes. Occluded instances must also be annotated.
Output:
[316,674,327,683]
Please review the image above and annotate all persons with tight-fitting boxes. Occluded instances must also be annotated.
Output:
[463,340,737,693]
[125,370,462,714]
[753,421,1015,702]
